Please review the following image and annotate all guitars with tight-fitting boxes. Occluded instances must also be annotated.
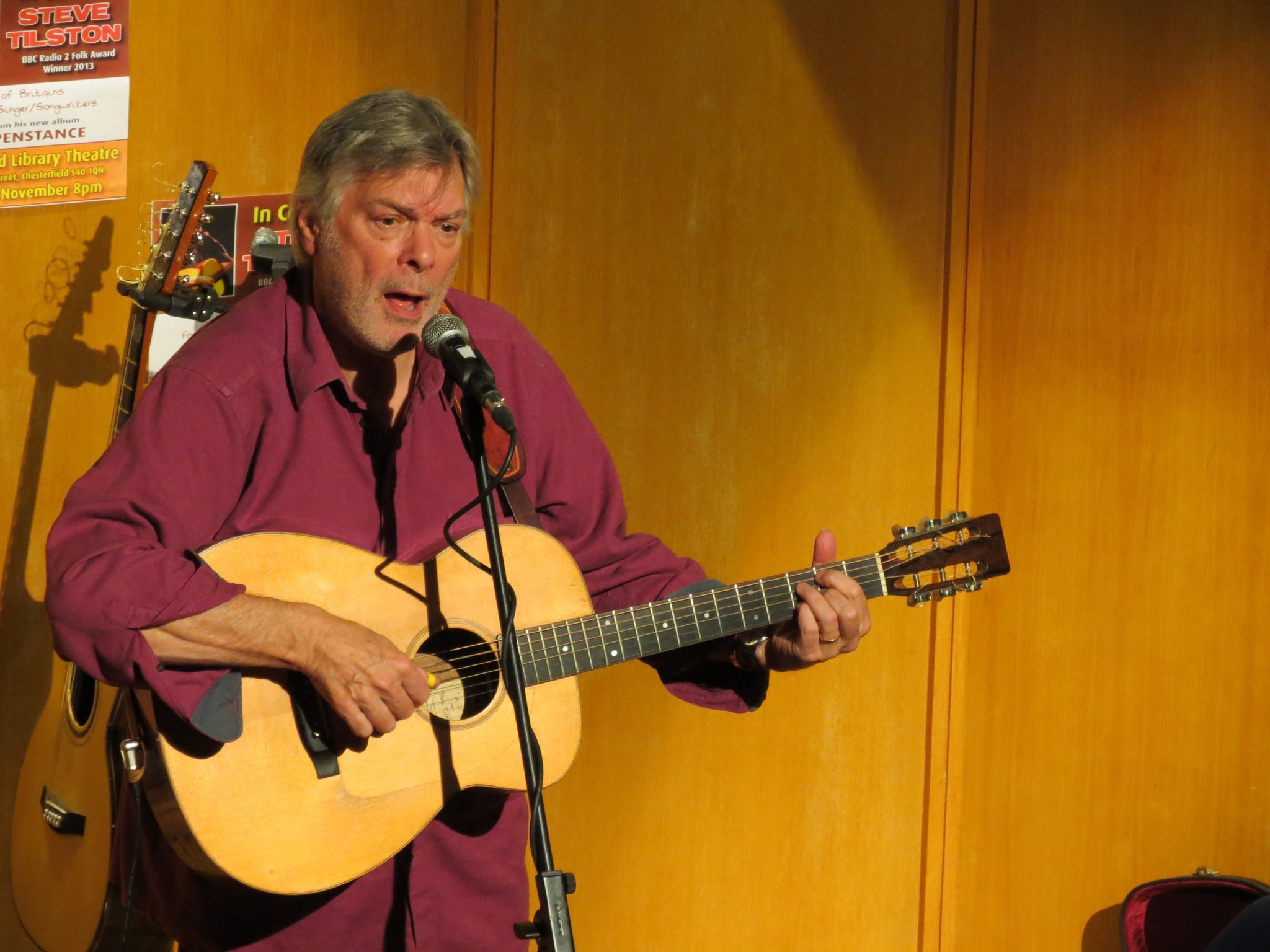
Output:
[117,510,1013,898]
[8,158,230,952]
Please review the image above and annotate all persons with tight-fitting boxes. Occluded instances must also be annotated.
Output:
[39,93,875,952]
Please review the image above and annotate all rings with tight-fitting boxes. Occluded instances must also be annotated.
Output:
[817,633,841,644]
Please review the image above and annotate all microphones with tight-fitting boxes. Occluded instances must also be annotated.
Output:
[421,313,516,436]
